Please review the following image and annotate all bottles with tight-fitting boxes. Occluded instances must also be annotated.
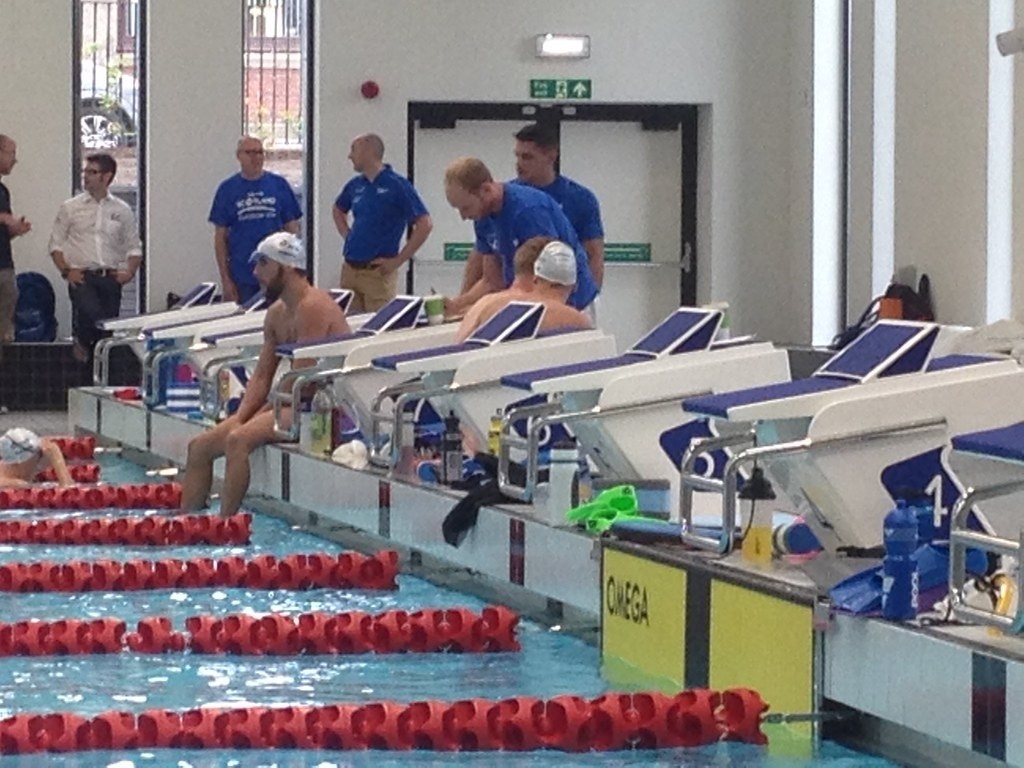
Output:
[488,408,504,457]
[549,435,579,522]
[403,408,414,448]
[440,410,462,484]
[301,401,312,451]
[740,468,775,566]
[311,382,331,453]
[882,500,919,619]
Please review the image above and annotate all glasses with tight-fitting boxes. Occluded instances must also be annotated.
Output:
[248,252,263,265]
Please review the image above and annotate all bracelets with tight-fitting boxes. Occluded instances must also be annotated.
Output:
[60,265,72,279]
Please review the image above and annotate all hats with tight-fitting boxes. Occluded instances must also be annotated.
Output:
[534,242,577,286]
[258,232,307,271]
[0,428,42,465]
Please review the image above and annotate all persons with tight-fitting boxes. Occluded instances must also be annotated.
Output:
[0,428,76,490]
[208,137,303,301]
[180,231,354,517]
[450,236,595,347]
[0,134,32,342]
[48,153,143,368]
[442,124,604,322]
[332,132,433,313]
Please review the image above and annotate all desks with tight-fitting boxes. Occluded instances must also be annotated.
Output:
[6,341,75,412]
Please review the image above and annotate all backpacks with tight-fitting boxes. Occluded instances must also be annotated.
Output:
[878,284,934,321]
[14,271,58,342]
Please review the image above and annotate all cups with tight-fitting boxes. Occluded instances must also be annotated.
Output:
[423,296,444,326]
